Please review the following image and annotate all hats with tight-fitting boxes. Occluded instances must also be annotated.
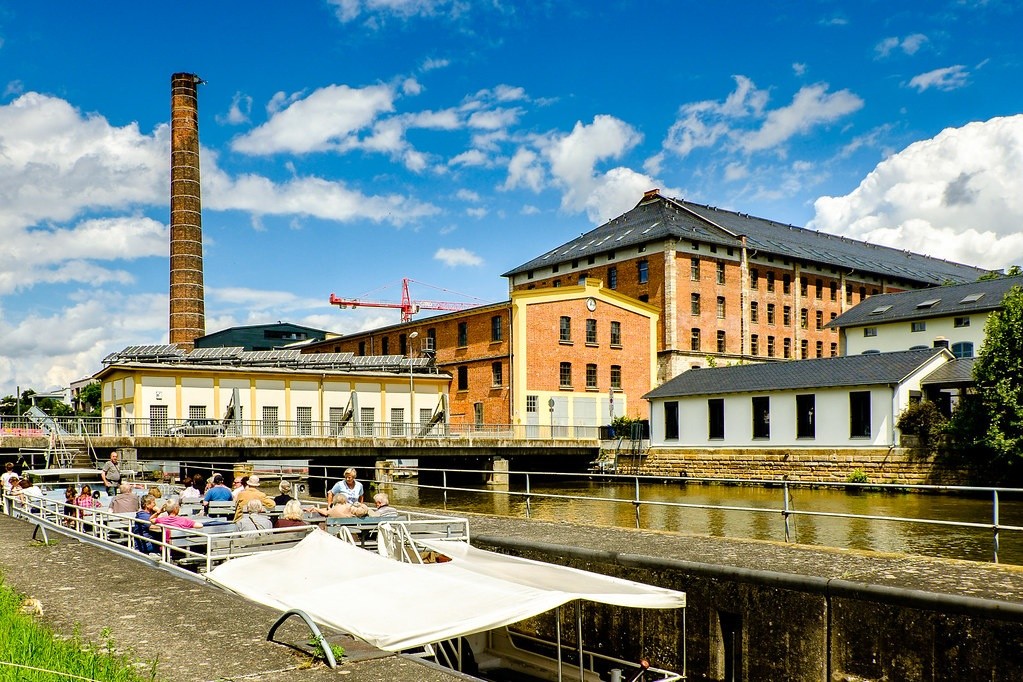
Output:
[279,480,291,495]
[246,475,260,486]
[214,475,224,483]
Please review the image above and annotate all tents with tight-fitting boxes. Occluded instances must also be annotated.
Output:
[203,528,686,682]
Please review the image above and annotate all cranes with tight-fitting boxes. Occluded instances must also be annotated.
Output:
[329,277,496,324]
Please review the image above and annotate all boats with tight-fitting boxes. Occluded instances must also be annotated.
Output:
[0,418,688,682]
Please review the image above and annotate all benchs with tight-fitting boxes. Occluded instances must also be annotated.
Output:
[19,490,407,566]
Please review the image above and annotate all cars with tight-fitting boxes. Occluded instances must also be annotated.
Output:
[164,418,226,438]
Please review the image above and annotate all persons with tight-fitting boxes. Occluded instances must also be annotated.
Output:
[274,480,296,506]
[234,475,276,522]
[109,483,140,513]
[327,468,364,510]
[101,452,122,496]
[0,462,46,513]
[276,493,398,542]
[231,499,273,548]
[62,484,102,528]
[133,473,249,555]
